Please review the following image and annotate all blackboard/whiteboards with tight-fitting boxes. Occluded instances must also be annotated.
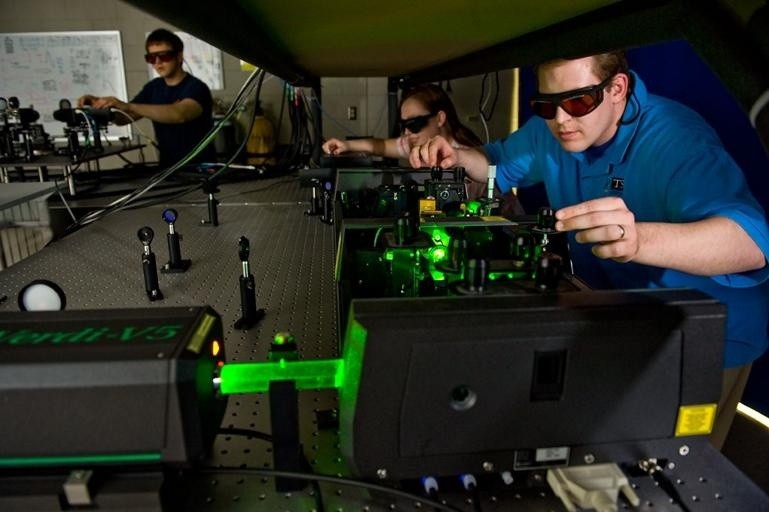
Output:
[0,31,134,146]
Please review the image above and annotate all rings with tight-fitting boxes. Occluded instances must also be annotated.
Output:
[617,224,626,239]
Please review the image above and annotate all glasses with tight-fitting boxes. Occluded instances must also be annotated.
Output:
[144,48,180,63]
[530,72,616,119]
[398,112,436,133]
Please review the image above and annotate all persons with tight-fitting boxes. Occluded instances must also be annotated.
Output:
[78,29,216,177]
[408,49,768,276]
[321,84,491,200]
